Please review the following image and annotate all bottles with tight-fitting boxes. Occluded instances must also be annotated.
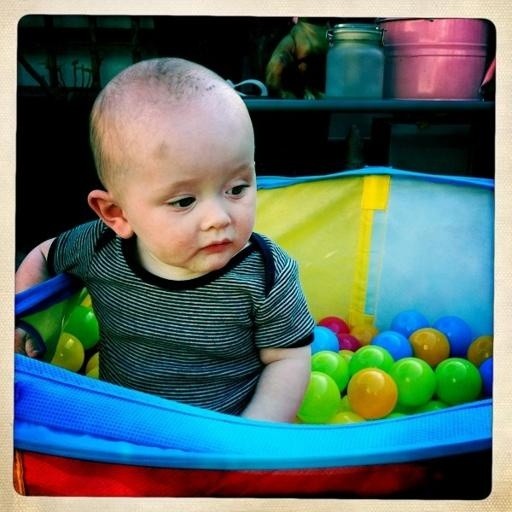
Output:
[321,24,387,101]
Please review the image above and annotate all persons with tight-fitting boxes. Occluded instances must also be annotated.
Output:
[267,17,366,172]
[16,56,315,423]
